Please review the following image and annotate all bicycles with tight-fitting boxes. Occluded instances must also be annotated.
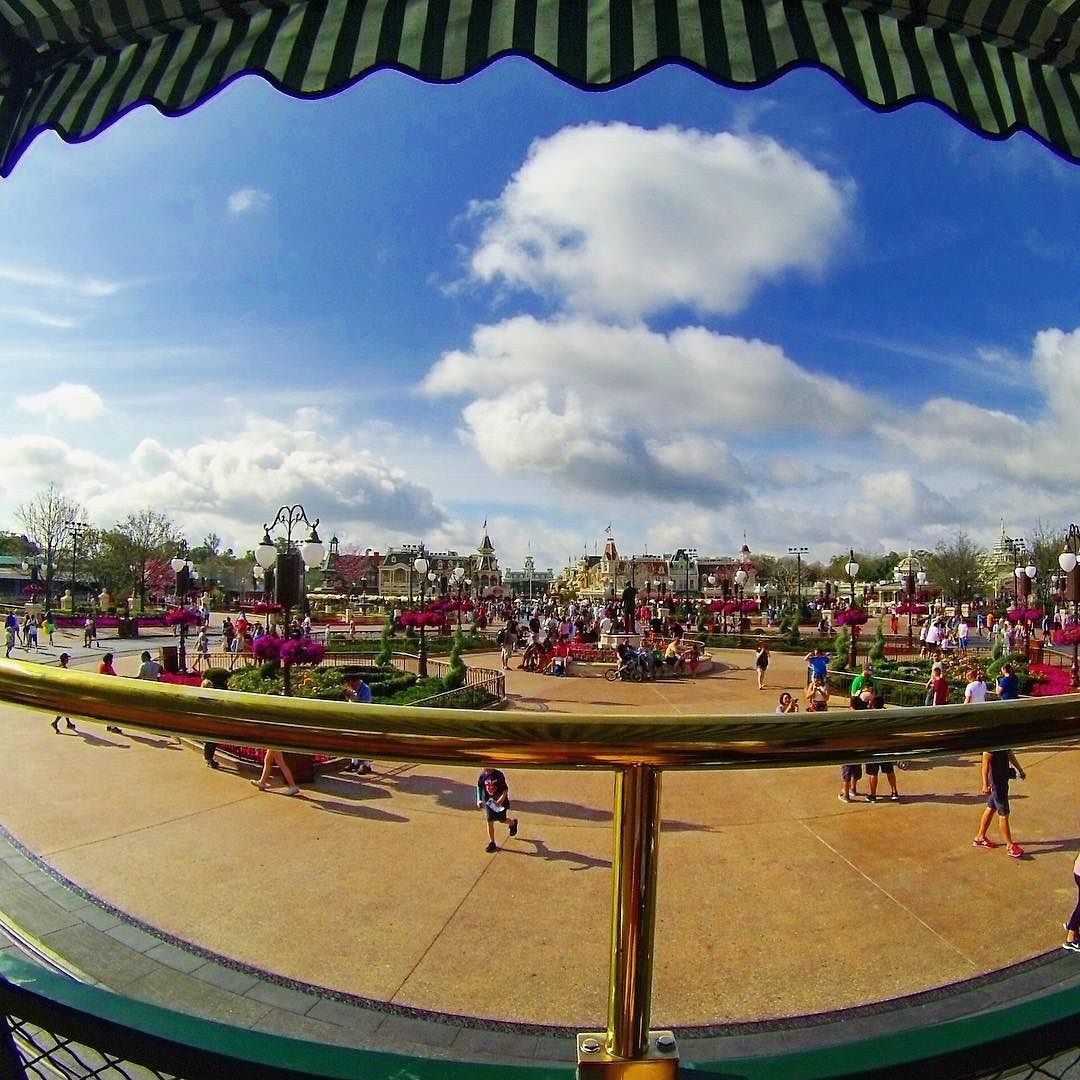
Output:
[603,654,646,683]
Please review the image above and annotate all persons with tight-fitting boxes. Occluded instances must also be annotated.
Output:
[4,579,1080,952]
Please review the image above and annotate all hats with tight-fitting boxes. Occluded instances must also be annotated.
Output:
[863,664,873,674]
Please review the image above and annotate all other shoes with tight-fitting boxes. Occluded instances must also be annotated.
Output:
[67,724,75,728]
[52,722,60,731]
[890,793,898,800]
[284,787,298,795]
[865,795,877,803]
[251,780,265,790]
[838,793,850,803]
[486,843,496,852]
[206,759,219,768]
[510,819,517,836]
[358,765,371,774]
[843,788,856,796]
[344,763,359,771]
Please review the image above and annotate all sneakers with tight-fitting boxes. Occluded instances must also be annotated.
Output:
[972,838,993,848]
[1063,940,1080,952]
[1006,845,1025,857]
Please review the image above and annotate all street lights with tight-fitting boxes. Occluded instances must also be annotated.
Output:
[844,547,861,672]
[410,545,437,684]
[893,556,926,649]
[1058,523,1080,689]
[62,521,87,619]
[1004,538,1025,606]
[788,547,809,608]
[735,563,748,633]
[1014,548,1037,662]
[170,538,194,675]
[21,548,48,604]
[253,502,326,701]
[448,562,471,634]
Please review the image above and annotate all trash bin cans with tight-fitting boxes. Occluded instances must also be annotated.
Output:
[162,646,178,673]
[118,617,138,640]
